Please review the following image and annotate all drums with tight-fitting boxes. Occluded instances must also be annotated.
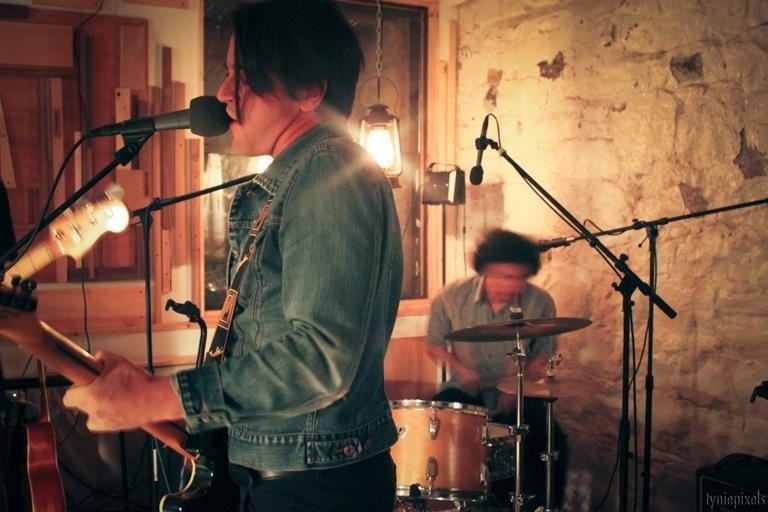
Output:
[487,422,516,481]
[388,399,489,502]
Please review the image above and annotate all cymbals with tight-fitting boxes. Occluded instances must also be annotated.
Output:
[496,377,603,398]
[444,318,592,342]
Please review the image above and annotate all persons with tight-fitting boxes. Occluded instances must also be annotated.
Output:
[426,229,559,511]
[63,1,406,512]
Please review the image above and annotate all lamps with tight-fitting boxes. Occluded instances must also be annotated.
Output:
[358,0,405,190]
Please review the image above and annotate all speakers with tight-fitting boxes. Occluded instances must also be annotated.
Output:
[695,454,768,512]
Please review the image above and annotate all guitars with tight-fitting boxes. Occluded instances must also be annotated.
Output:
[1,183,130,289]
[0,275,240,512]
[27,358,66,512]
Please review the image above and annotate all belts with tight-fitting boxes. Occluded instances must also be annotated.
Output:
[259,471,300,481]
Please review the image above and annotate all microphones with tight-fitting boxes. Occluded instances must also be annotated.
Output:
[536,236,576,252]
[470,115,489,185]
[90,95,231,137]
